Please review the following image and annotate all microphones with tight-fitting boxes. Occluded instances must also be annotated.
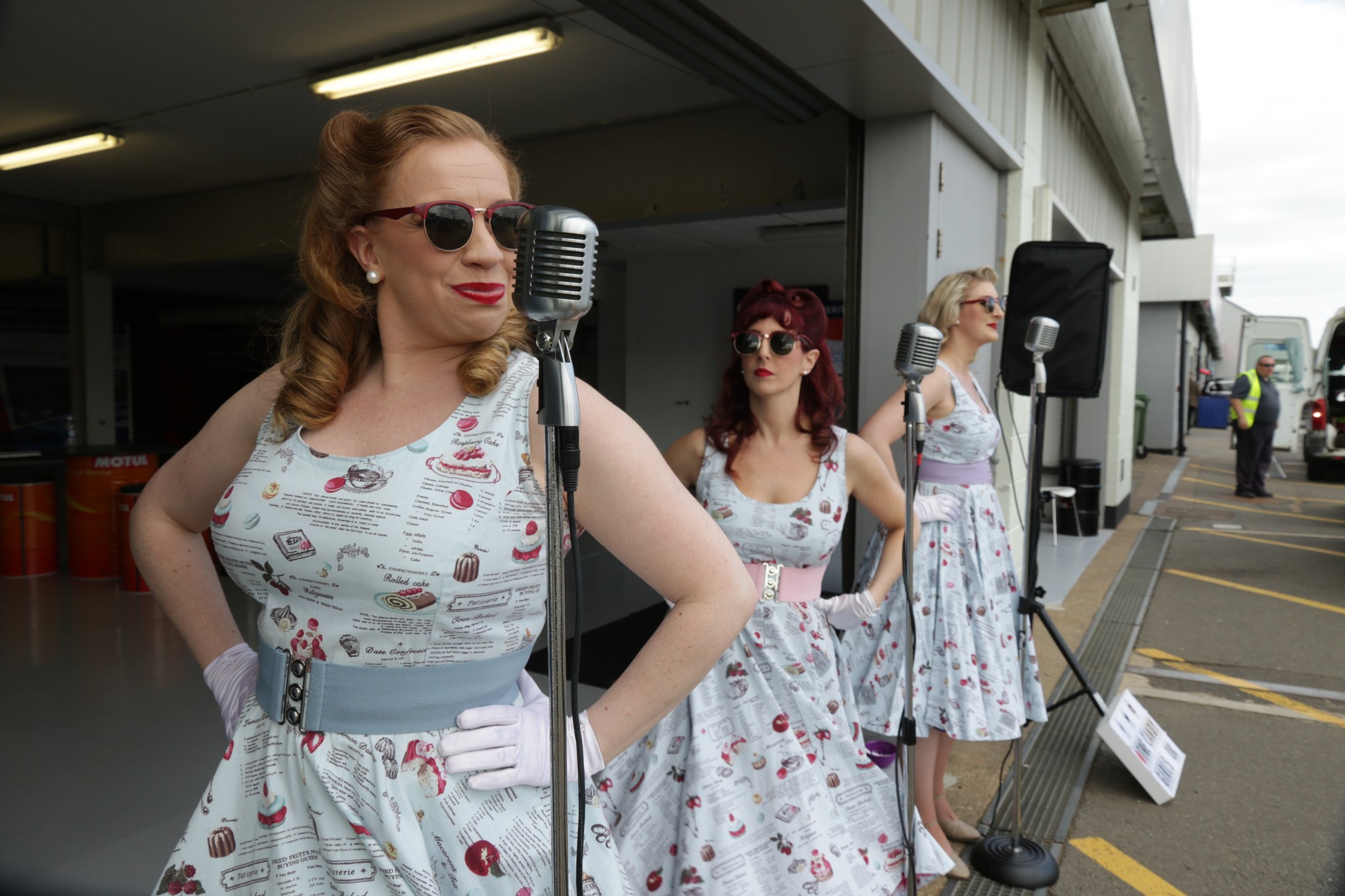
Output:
[896,321,945,378]
[1025,316,1059,353]
[513,206,598,322]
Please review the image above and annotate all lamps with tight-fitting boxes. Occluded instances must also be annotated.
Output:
[0,125,128,172]
[308,16,565,99]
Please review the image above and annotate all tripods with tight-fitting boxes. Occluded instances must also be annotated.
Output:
[1022,394,1106,714]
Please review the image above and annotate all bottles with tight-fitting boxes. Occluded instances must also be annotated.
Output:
[65,415,76,446]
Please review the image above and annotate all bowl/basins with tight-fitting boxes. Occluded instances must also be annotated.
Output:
[865,741,896,768]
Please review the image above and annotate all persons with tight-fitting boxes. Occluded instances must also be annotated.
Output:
[1230,355,1280,498]
[859,271,1050,884]
[130,107,758,896]
[586,281,956,896]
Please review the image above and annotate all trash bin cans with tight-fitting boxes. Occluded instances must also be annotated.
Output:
[1057,458,1103,535]
[1135,390,1151,445]
[1132,399,1146,461]
[1197,395,1229,428]
[123,486,213,595]
[65,444,158,581]
[0,452,58,582]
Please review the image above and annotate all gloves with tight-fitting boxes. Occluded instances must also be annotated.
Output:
[913,494,962,523]
[814,591,880,631]
[202,643,259,738]
[438,669,606,790]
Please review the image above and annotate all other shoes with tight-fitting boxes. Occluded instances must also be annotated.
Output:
[942,821,981,842]
[1236,488,1255,498]
[1252,488,1273,498]
[946,851,970,879]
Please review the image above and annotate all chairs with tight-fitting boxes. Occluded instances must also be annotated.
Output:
[1042,485,1083,545]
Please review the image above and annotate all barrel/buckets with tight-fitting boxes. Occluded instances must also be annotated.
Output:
[118,482,215,594]
[0,475,61,578]
[1057,458,1101,536]
[64,453,158,580]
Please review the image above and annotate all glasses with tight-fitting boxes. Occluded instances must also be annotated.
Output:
[958,296,1007,314]
[355,204,529,253]
[731,331,813,357]
[1260,363,1275,368]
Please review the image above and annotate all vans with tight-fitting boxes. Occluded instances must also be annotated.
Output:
[1229,307,1345,484]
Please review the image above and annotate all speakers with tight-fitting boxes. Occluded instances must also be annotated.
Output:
[1000,241,1113,396]
